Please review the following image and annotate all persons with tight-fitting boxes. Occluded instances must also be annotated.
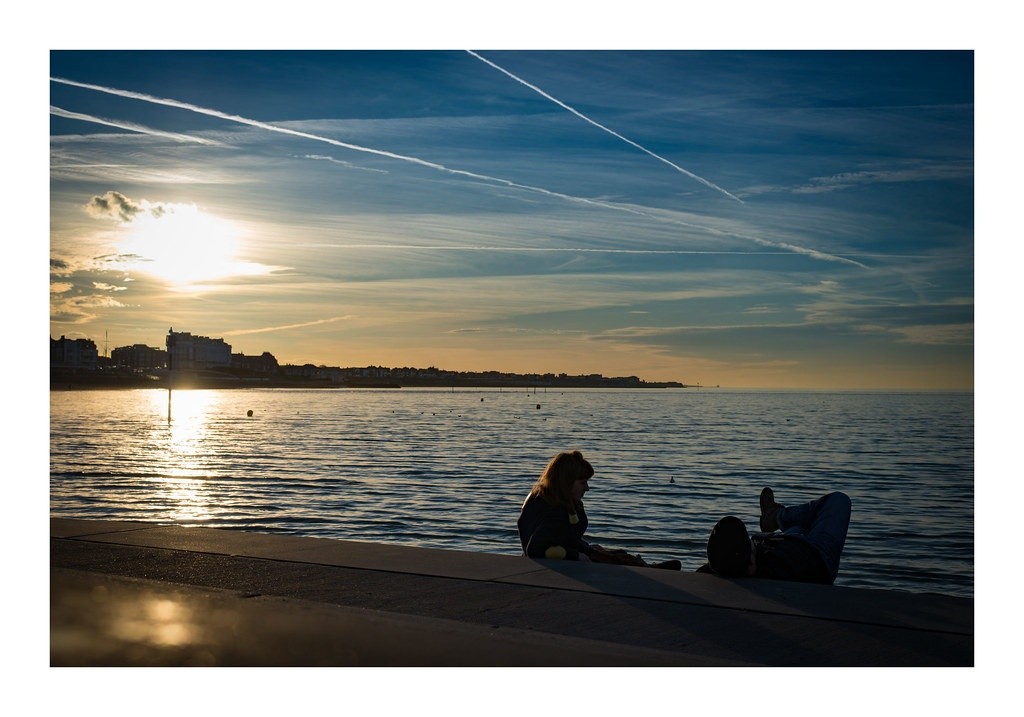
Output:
[694,487,852,587]
[516,449,684,572]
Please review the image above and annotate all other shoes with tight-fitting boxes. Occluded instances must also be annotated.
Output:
[663,560,681,570]
[760,487,783,533]
